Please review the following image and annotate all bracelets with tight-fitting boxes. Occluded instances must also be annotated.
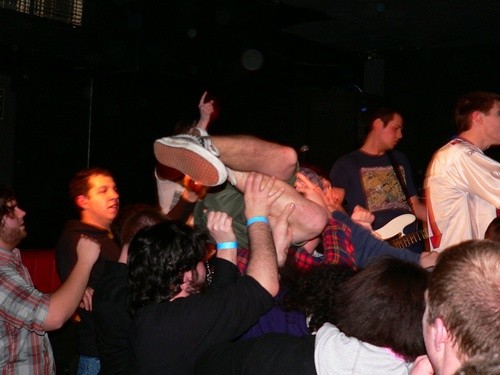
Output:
[245,216,269,226]
[215,242,236,250]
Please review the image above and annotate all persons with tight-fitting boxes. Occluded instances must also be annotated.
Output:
[2,92,499,375]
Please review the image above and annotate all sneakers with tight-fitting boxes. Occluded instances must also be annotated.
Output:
[155,128,227,187]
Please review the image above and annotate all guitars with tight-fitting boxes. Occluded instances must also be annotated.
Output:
[373,214,425,249]
